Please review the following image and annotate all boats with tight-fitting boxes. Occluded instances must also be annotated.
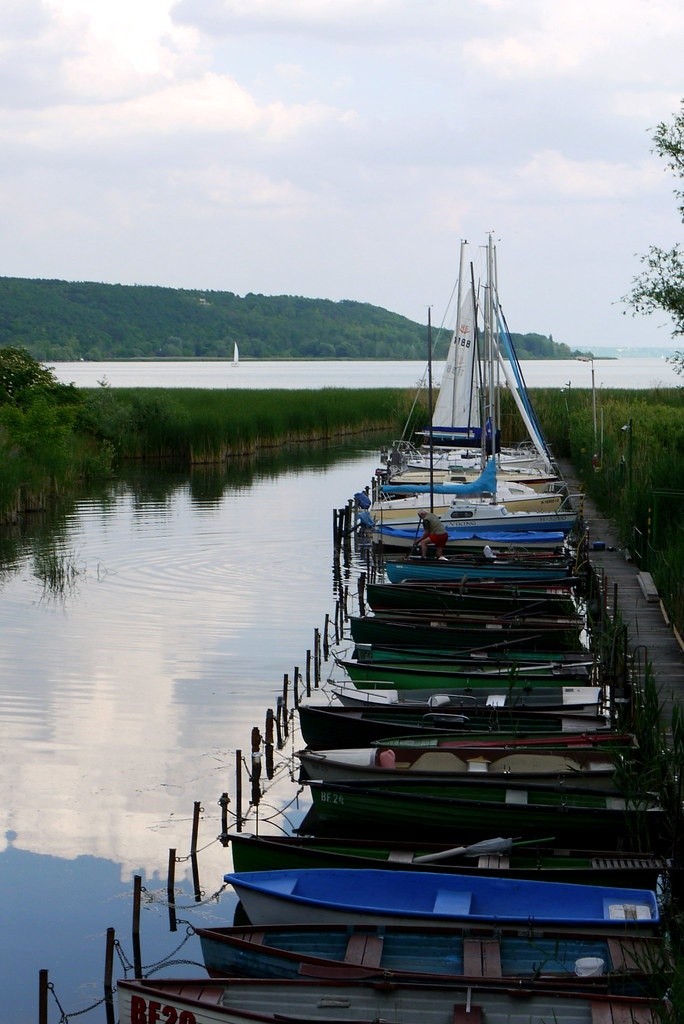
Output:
[196,923,677,998]
[224,868,660,925]
[298,694,612,751]
[338,240,601,689]
[304,780,668,833]
[228,835,672,890]
[117,979,673,1024]
[370,731,640,753]
[327,679,607,707]
[293,748,621,792]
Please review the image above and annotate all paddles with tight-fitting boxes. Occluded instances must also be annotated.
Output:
[452,634,543,655]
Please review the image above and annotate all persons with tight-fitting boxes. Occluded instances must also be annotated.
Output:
[414,509,448,559]
